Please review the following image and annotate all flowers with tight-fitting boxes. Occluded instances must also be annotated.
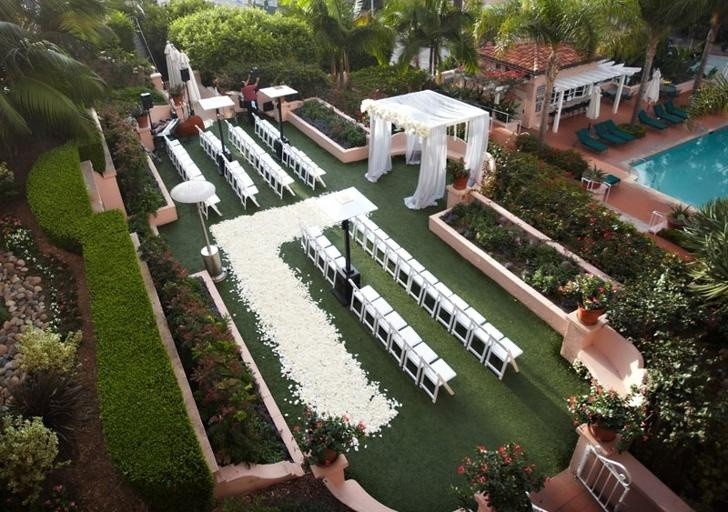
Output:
[296,412,369,467]
[568,376,650,454]
[560,270,613,311]
[457,439,546,511]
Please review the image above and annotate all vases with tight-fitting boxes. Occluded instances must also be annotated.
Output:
[322,448,337,461]
[575,305,604,325]
[587,420,616,441]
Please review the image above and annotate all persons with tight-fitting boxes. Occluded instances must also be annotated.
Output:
[239,72,261,125]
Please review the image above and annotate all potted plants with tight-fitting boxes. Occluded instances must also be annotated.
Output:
[663,202,690,231]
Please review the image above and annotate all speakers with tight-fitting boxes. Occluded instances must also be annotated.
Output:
[141,93,153,108]
[180,68,190,81]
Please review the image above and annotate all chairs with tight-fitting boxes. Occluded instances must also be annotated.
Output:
[300,214,524,405]
[652,102,683,123]
[574,128,607,155]
[600,174,621,191]
[162,113,328,220]
[600,84,634,106]
[664,101,687,118]
[593,123,624,145]
[637,108,669,130]
[603,119,634,141]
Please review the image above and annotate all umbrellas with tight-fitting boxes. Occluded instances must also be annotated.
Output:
[586,84,602,132]
[164,40,174,84]
[170,44,186,90]
[177,50,202,116]
[643,68,663,113]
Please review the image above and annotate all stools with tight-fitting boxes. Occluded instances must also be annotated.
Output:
[548,99,592,124]
